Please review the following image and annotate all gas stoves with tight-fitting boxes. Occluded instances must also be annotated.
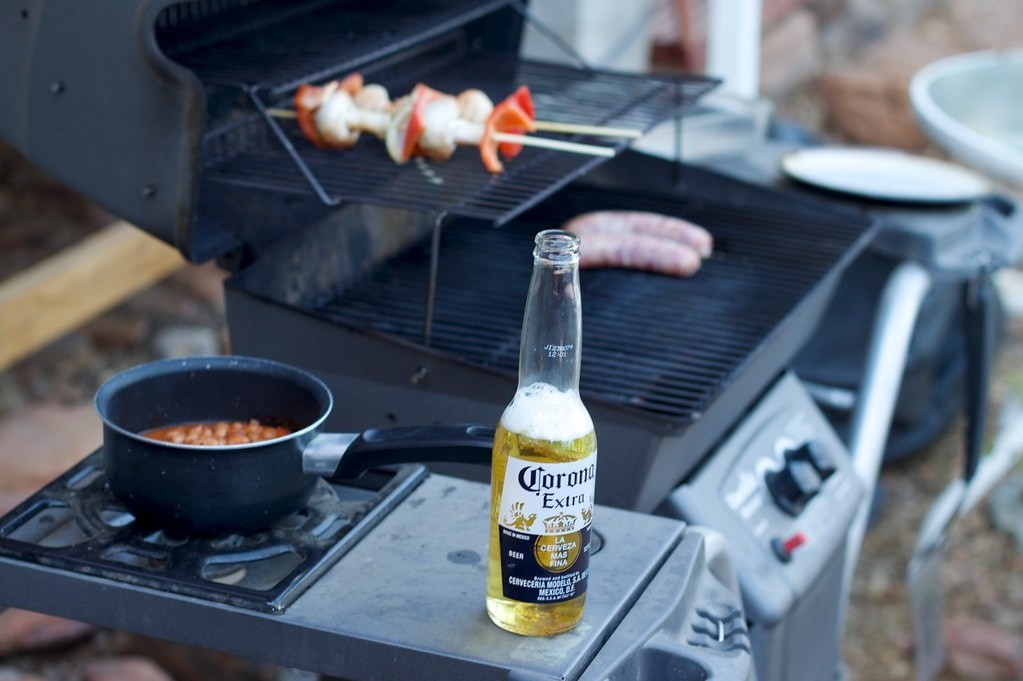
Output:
[0,445,426,614]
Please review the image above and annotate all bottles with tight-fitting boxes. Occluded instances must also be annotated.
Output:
[485,229,598,637]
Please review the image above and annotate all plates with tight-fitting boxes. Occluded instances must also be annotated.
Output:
[784,145,989,201]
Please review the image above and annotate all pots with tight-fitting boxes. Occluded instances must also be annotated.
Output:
[93,355,495,532]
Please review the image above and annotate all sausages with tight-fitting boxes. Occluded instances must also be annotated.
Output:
[564,210,714,278]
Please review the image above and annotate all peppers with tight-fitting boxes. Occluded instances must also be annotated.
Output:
[295,73,535,173]
